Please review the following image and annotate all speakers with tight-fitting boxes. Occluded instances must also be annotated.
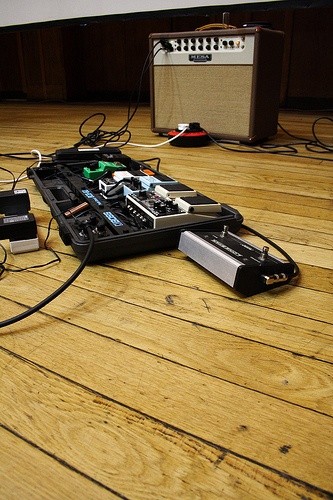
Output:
[148,27,282,144]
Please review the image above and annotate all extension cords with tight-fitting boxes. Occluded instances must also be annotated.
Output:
[5,211,39,254]
